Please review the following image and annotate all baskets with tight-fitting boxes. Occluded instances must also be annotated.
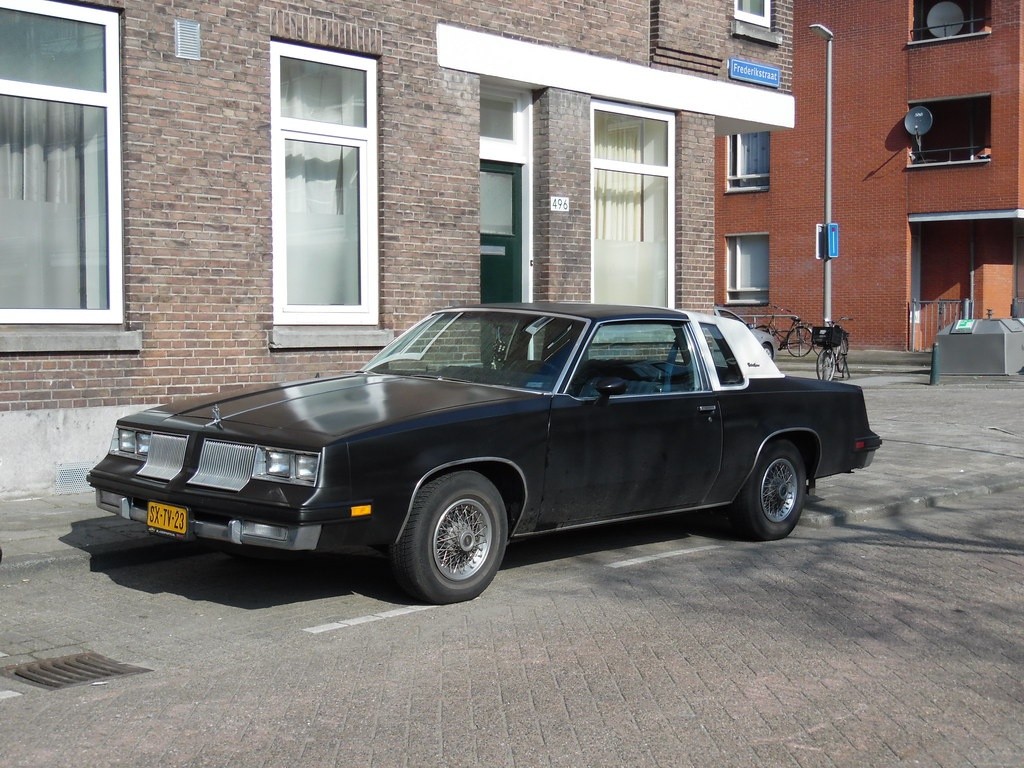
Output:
[811,327,842,347]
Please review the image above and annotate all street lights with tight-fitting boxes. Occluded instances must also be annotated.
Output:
[810,24,834,383]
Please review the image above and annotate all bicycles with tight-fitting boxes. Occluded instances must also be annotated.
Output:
[812,317,853,382]
[755,303,815,357]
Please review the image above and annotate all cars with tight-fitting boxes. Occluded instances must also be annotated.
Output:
[713,305,776,365]
[85,299,886,603]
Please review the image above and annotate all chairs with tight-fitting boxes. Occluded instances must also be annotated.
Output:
[507,360,684,398]
